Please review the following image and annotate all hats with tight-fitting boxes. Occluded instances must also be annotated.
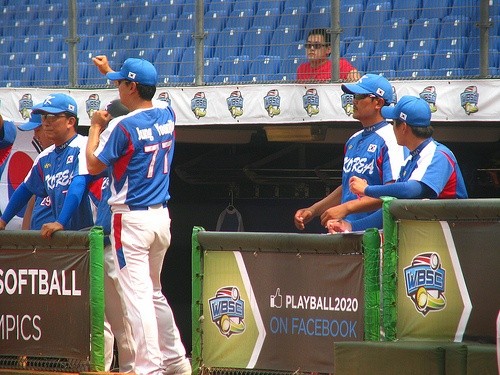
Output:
[17,113,42,131]
[37,93,78,115]
[106,57,157,86]
[341,73,393,104]
[381,96,431,127]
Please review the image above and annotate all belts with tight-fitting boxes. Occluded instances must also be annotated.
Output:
[104,236,111,248]
[129,202,168,211]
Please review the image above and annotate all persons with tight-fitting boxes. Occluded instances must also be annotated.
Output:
[295,30,361,82]
[326,94,469,234]
[1,92,134,375]
[84,55,196,375]
[294,73,414,233]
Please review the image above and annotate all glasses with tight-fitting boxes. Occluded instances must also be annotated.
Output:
[353,94,377,100]
[305,42,325,49]
[118,80,137,86]
[40,113,69,122]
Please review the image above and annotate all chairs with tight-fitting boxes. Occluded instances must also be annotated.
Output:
[0,0,500,87]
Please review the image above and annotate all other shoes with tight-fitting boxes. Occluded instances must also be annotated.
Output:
[163,358,192,375]
